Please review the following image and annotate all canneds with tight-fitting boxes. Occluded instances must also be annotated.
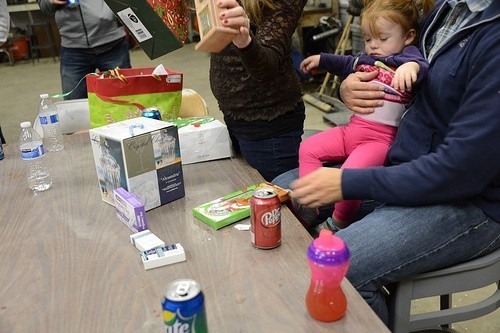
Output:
[65,0,79,8]
[249,188,281,249]
[161,278,207,333]
[142,107,161,121]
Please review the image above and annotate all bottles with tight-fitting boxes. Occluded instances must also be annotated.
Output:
[305,229,351,322]
[38,94,66,153]
[19,121,53,192]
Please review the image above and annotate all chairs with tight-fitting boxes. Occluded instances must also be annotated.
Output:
[179,88,208,120]
[33,99,90,135]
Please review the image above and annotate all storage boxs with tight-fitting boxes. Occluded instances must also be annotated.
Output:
[142,243,184,271]
[192,181,290,229]
[89,118,185,211]
[113,187,148,234]
[129,232,165,253]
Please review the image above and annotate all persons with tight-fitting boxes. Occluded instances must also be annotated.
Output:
[209,0,308,183]
[0,0,10,160]
[271,0,499,328]
[36,0,132,101]
[299,0,429,237]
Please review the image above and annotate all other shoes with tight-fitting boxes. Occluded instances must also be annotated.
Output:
[315,217,341,235]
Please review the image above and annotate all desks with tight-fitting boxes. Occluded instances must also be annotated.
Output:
[8,4,41,57]
[291,6,329,55]
[0,134,393,333]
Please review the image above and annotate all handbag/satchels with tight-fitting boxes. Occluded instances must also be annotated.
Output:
[86,65,184,128]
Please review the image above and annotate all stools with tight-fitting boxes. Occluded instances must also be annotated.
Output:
[371,248,500,333]
[27,22,59,65]
